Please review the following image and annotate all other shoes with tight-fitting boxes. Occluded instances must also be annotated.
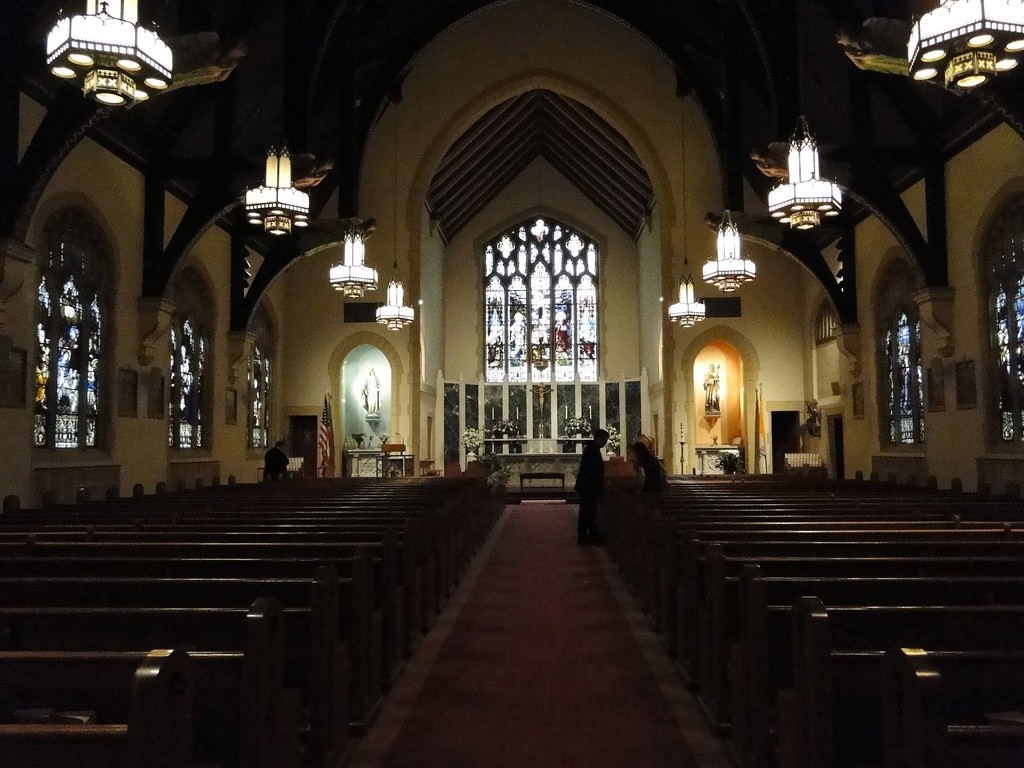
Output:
[589,533,604,541]
[577,534,597,544]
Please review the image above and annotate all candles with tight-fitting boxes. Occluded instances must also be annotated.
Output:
[565,406,569,420]
[589,405,592,420]
[517,407,519,420]
[492,407,494,419]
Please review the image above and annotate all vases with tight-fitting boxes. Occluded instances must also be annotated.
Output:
[606,446,615,454]
[467,447,476,455]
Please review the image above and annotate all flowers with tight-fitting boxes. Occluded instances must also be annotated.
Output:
[461,425,486,447]
[604,424,622,449]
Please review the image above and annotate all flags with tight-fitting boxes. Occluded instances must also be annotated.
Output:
[318,394,331,476]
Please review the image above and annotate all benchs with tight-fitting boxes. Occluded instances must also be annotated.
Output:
[520,473,565,488]
[1,472,505,767]
[598,474,1024,768]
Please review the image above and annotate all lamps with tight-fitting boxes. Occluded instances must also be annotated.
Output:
[907,0,1024,90]
[330,0,378,298]
[244,0,311,237]
[375,82,415,331]
[45,0,173,106]
[703,0,756,293]
[668,75,706,327]
[768,0,842,230]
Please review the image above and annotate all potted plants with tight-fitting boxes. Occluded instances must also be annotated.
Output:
[715,452,744,475]
[351,433,365,450]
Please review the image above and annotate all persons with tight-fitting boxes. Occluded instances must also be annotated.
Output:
[632,442,668,495]
[703,363,720,412]
[529,387,554,413]
[262,441,289,481]
[573,429,611,546]
[361,368,379,416]
